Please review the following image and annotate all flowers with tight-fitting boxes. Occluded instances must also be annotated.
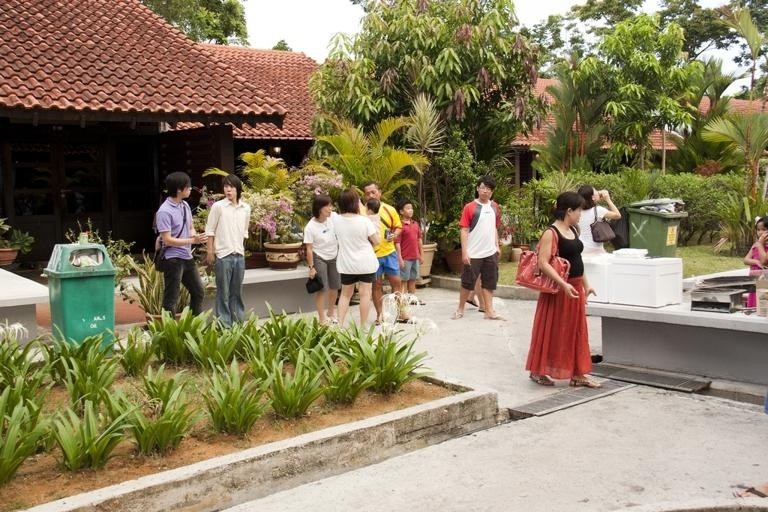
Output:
[205,167,347,242]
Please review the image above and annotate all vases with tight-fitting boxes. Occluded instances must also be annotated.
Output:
[512,242,530,252]
[263,240,301,270]
[510,248,522,262]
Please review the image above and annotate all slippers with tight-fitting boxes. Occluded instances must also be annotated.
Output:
[451,310,463,320]
[410,298,426,306]
[466,297,485,312]
[374,317,387,325]
[484,311,506,321]
[396,314,419,324]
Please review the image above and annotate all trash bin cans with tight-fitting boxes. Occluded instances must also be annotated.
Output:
[623,198,689,259]
[43,243,118,360]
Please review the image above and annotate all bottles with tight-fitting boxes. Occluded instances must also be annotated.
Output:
[385,228,390,239]
[756,270,767,317]
[77,232,90,244]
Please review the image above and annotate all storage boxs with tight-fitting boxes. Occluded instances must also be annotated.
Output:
[608,248,684,306]
[581,252,614,303]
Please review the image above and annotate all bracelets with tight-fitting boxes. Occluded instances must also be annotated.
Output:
[308,265,315,268]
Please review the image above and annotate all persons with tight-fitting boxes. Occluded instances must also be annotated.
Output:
[156,171,207,314]
[395,201,426,306]
[334,189,381,332]
[578,185,622,253]
[363,182,410,326]
[467,275,485,313]
[744,217,768,306]
[206,174,251,324]
[365,198,381,242]
[451,175,504,321]
[304,196,342,324]
[528,192,601,389]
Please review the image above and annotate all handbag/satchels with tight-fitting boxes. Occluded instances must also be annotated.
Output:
[590,205,616,242]
[514,228,571,295]
[306,273,324,293]
[152,245,166,272]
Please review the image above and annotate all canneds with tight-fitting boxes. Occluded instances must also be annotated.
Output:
[384,228,391,239]
[79,232,89,245]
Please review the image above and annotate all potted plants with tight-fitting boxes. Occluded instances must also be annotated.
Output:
[524,178,560,252]
[402,91,449,277]
[442,219,463,274]
[0,217,36,266]
[124,248,190,325]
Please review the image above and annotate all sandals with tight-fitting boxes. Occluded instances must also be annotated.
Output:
[568,374,602,389]
[529,371,555,387]
